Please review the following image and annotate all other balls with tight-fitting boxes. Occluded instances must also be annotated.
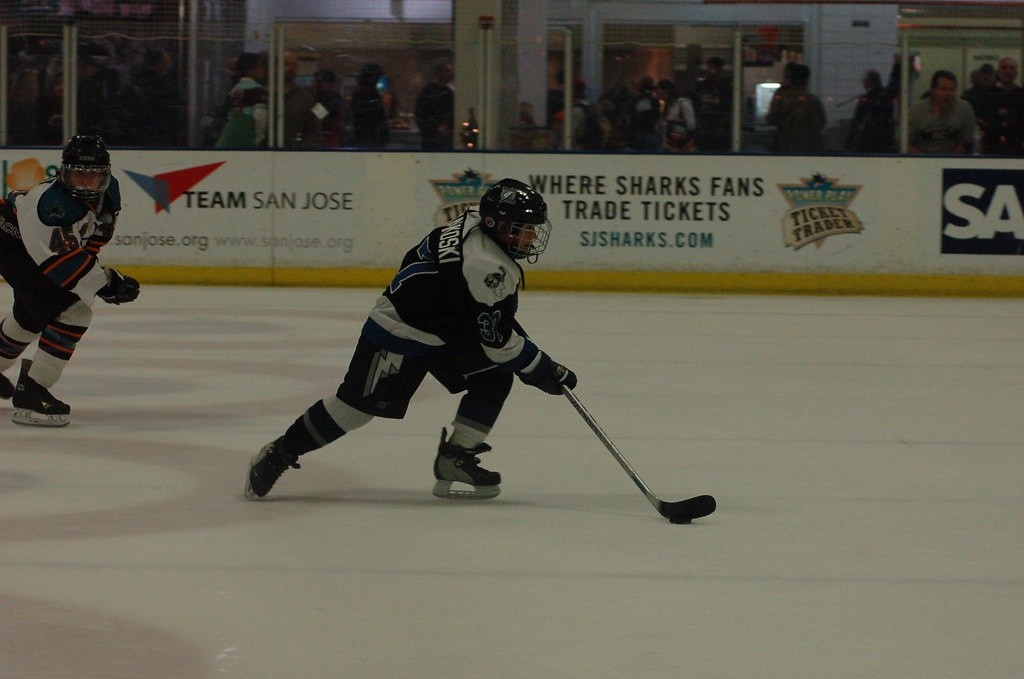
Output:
[668,516,692,524]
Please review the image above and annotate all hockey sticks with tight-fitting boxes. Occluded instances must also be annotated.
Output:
[512,316,717,520]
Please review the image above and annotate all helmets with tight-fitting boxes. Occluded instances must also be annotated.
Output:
[59,135,113,200]
[480,177,548,245]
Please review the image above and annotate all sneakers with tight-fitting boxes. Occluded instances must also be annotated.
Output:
[245,434,300,499]
[0,372,14,400]
[11,358,71,427]
[433,427,501,499]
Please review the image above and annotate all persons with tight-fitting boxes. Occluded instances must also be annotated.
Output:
[548,57,1024,160]
[39,31,400,152]
[414,53,459,152]
[245,178,577,498]
[0,135,141,427]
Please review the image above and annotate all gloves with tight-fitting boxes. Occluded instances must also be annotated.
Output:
[544,360,578,396]
[95,264,140,305]
